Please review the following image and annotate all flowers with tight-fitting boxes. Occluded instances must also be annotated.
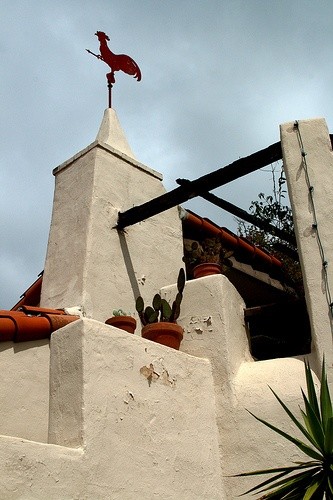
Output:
[182,239,234,272]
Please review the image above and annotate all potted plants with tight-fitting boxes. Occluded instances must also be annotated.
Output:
[104,309,137,335]
[136,268,186,351]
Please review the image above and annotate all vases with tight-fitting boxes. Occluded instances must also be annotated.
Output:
[193,263,224,279]
